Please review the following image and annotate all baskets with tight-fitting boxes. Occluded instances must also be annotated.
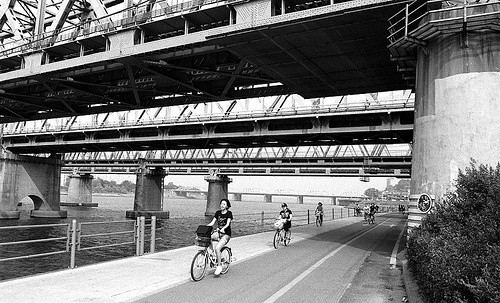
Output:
[273,222,283,230]
[195,235,211,247]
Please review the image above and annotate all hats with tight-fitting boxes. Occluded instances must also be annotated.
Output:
[318,202,323,205]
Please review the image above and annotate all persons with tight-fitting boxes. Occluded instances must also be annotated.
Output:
[398,204,405,215]
[206,199,233,276]
[356,202,379,224]
[315,202,324,225]
[277,203,292,241]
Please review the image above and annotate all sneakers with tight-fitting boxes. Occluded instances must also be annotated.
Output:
[208,250,214,255]
[214,266,222,276]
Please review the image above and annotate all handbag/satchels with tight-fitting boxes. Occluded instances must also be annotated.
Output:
[194,225,214,237]
[274,219,284,229]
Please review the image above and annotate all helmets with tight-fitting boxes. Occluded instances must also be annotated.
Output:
[282,203,287,208]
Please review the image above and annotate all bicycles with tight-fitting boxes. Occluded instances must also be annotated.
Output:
[314,211,325,227]
[355,208,375,225]
[273,218,294,249]
[190,235,233,282]
